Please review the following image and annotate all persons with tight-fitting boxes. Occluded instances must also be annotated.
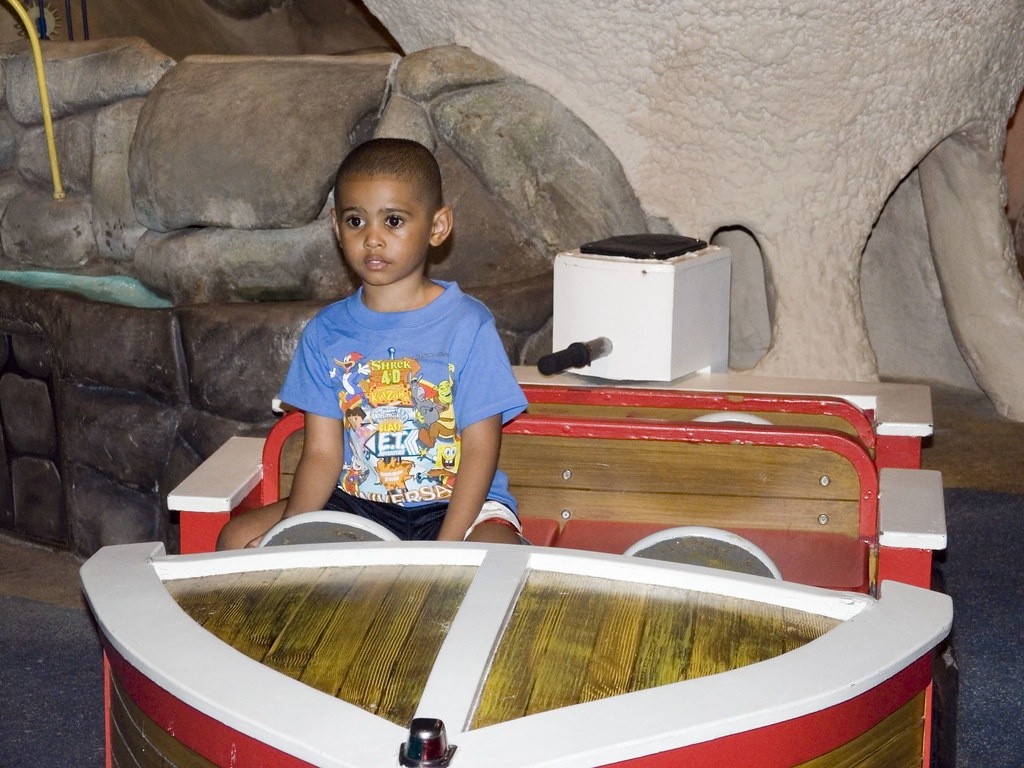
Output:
[214,137,528,553]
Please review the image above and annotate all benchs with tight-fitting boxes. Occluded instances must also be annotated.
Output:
[512,366,934,471]
[167,413,947,598]
